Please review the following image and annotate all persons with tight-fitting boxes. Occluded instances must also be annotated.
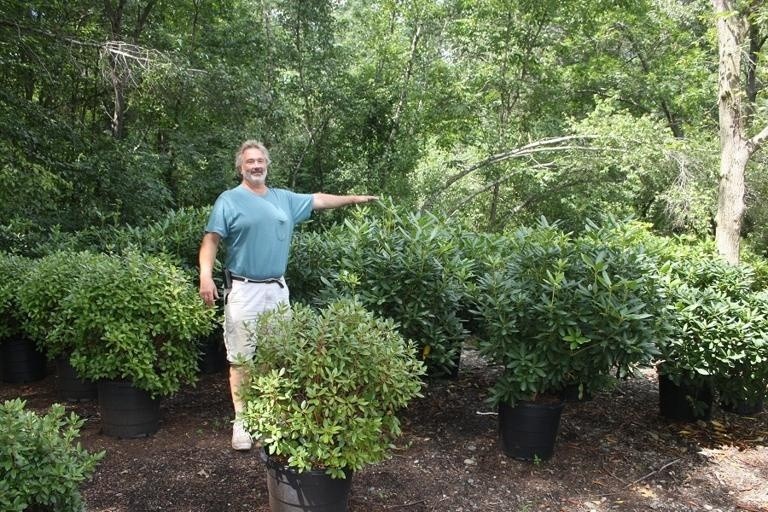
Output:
[200,140,380,450]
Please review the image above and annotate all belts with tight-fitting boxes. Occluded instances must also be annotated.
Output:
[231,276,284,287]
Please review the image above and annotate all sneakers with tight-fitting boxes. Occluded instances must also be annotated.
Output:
[232,420,253,451]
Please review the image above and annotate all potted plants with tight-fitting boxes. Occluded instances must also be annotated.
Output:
[81,264,196,435]
[429,266,467,377]
[554,233,621,403]
[655,275,714,420]
[483,250,566,462]
[249,300,423,509]
[39,260,101,398]
[711,290,766,414]
[4,253,37,385]
